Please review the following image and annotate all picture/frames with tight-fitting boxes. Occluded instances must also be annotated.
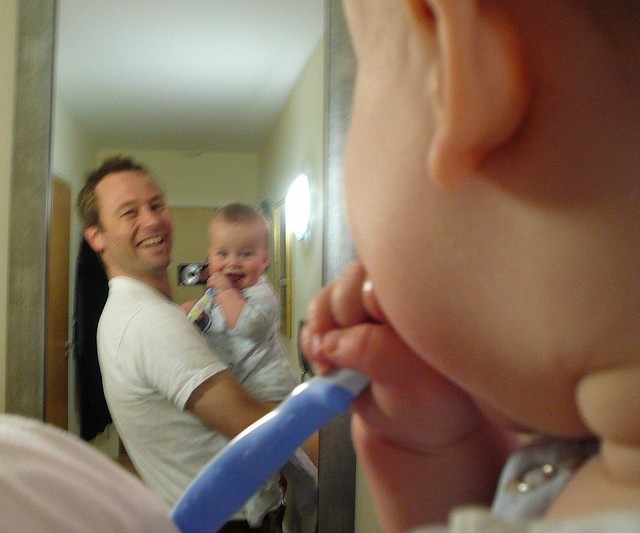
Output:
[273,197,286,340]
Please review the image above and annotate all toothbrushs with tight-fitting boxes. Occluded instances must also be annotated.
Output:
[168,368,370,528]
[186,273,242,325]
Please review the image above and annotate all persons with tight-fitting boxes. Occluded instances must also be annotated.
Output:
[302,1,639,533]
[182,203,304,529]
[78,154,277,533]
